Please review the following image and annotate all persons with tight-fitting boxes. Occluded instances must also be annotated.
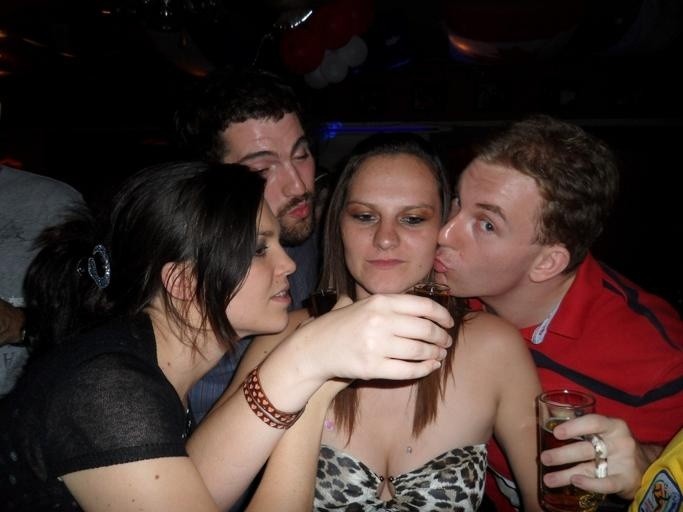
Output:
[427,112,683,512]
[171,61,326,418]
[0,157,356,510]
[203,132,586,512]
[0,159,93,412]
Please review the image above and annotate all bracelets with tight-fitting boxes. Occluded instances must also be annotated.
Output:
[240,372,303,431]
[9,304,31,348]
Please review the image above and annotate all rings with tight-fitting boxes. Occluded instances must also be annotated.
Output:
[590,432,608,464]
[594,458,608,483]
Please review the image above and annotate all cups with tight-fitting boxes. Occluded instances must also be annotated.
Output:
[534,389,599,512]
[410,282,450,310]
